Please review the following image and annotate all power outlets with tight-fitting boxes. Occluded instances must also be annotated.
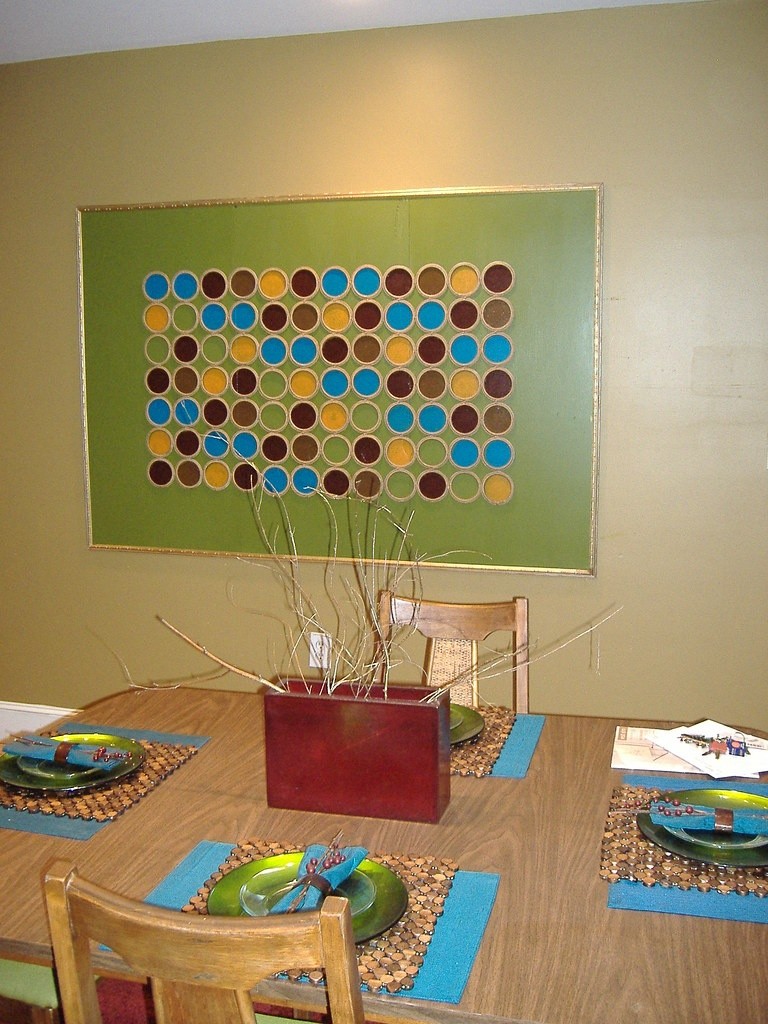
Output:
[309,631,331,671]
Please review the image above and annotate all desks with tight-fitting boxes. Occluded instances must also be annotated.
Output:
[0,686,768,1024]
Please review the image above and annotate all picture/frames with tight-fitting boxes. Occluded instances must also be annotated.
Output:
[72,182,606,579]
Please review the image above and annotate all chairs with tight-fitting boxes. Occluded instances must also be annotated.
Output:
[373,590,530,714]
[45,858,364,1024]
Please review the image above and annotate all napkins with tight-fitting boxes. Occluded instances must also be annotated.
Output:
[3,735,131,775]
[269,844,370,915]
[648,800,768,837]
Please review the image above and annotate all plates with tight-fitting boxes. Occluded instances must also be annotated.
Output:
[0,732,146,792]
[661,825,768,849]
[450,708,463,730]
[18,756,103,780]
[240,862,377,918]
[207,852,408,943]
[638,789,768,866]
[449,703,485,744]
